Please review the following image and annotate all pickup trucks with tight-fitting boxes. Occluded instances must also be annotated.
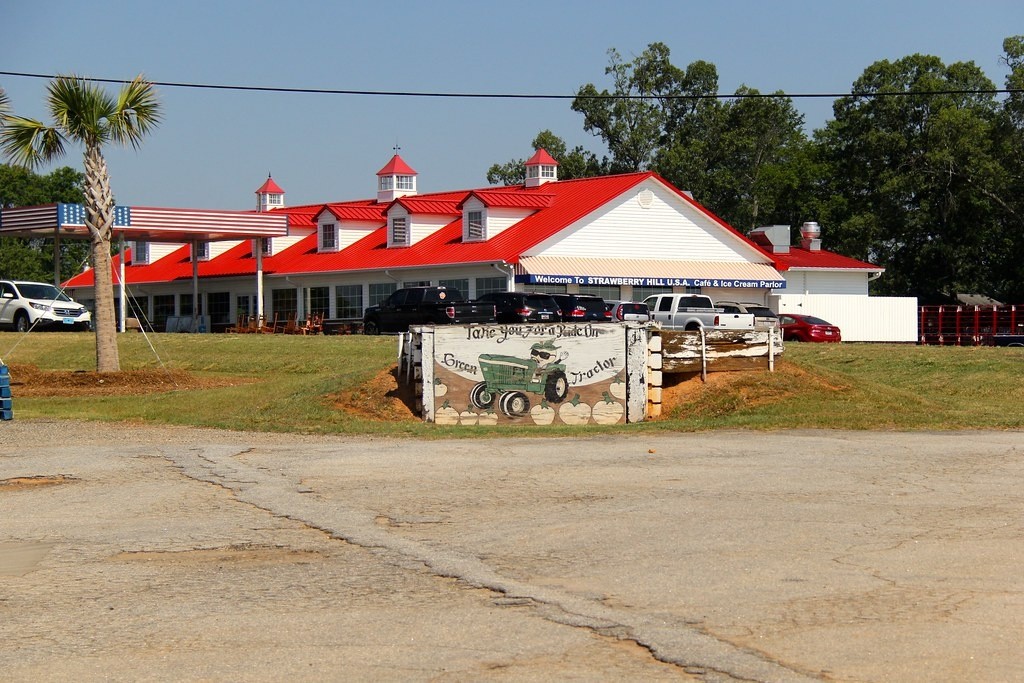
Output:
[642,293,755,331]
[363,286,498,335]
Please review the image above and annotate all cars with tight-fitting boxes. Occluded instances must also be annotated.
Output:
[775,314,841,343]
[985,324,1024,347]
[474,292,562,324]
[603,299,649,322]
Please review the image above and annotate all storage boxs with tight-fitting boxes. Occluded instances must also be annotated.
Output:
[0,365,12,420]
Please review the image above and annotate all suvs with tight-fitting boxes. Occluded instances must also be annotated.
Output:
[0,280,91,332]
[546,293,613,322]
[713,300,780,334]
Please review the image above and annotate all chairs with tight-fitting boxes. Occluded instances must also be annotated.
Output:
[225,312,324,336]
